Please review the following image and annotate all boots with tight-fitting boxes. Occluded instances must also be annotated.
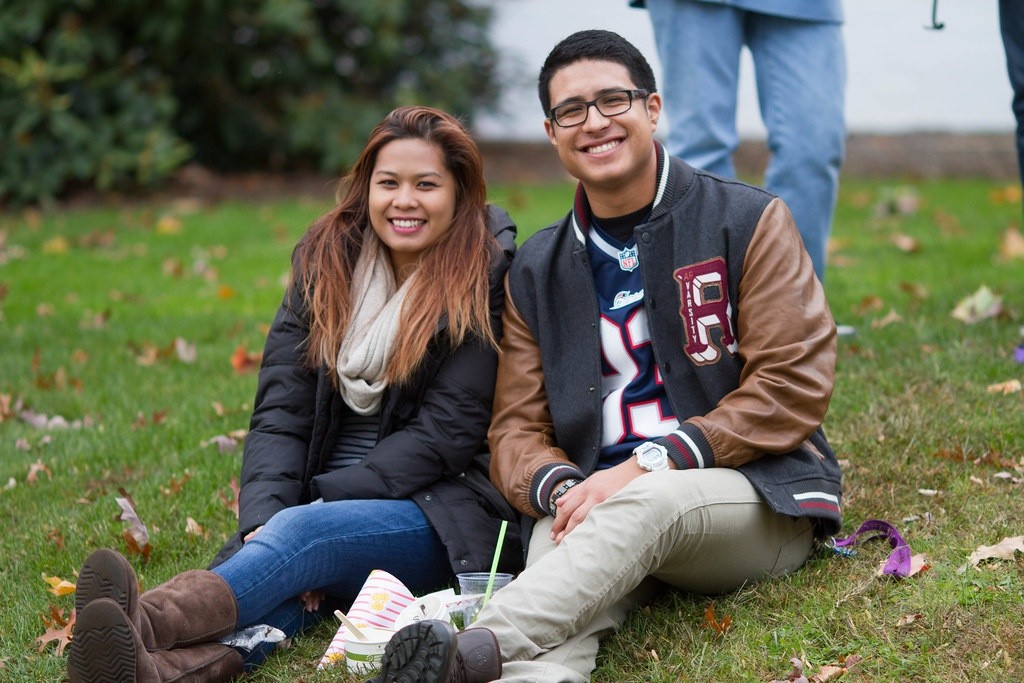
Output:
[366,618,504,683]
[66,547,245,683]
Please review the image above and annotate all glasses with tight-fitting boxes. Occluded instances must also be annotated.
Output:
[549,88,651,129]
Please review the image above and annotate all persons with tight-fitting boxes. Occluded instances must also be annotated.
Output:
[630,0,854,335]
[379,29,841,683]
[66,105,518,683]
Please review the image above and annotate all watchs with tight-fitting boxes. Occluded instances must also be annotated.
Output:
[632,441,670,472]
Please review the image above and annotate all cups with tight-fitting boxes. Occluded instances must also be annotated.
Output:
[456,572,515,630]
[343,627,393,674]
[393,593,459,634]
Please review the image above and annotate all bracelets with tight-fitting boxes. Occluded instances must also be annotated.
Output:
[549,479,581,517]
